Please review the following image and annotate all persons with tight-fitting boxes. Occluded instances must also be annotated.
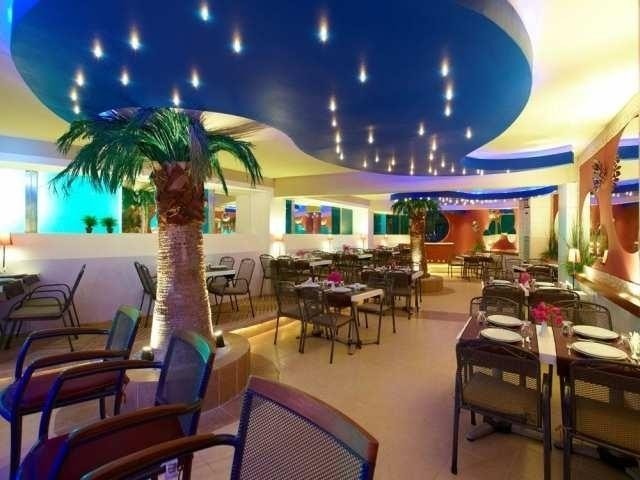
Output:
[491,233,515,250]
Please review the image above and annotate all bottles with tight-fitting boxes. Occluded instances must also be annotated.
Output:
[478,311,485,327]
[563,326,570,337]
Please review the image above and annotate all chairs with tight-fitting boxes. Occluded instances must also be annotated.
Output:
[258,242,428,365]
[131,259,160,313]
[206,256,235,310]
[79,377,382,480]
[17,330,215,480]
[208,258,255,325]
[452,340,554,480]
[448,243,594,323]
[140,264,158,327]
[2,303,143,440]
[552,300,620,400]
[3,268,85,353]
[10,263,87,338]
[462,294,526,383]
[562,355,640,480]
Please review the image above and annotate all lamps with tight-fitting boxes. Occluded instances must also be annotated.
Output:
[0,230,13,273]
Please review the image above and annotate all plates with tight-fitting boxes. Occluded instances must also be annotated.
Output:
[330,284,367,293]
[572,325,628,361]
[480,315,524,344]
